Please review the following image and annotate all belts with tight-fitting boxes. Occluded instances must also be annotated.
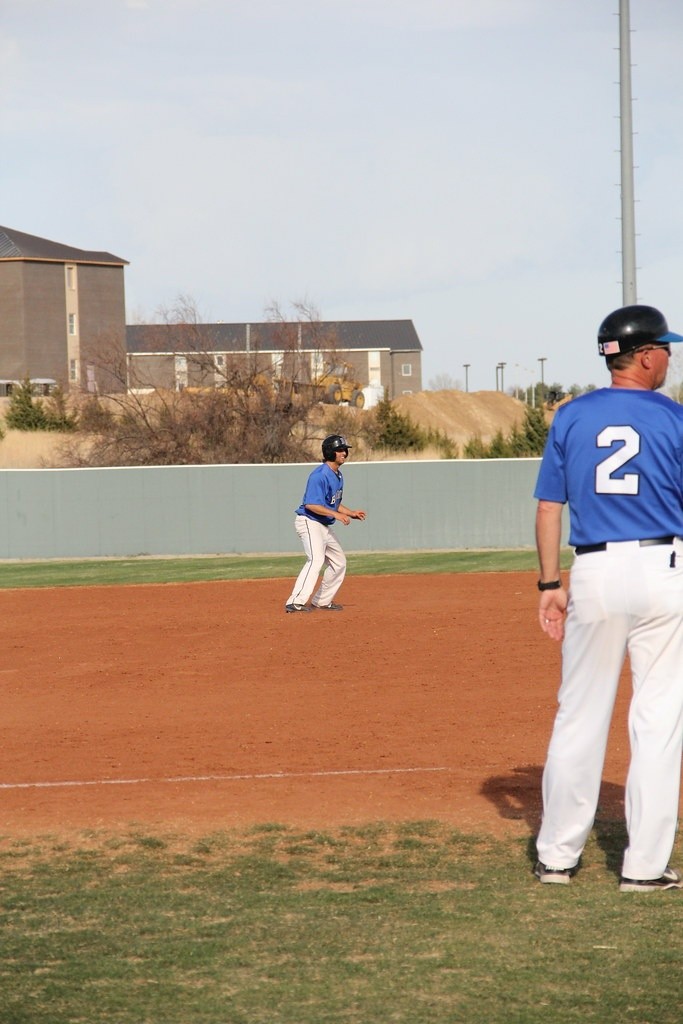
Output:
[576,536,673,555]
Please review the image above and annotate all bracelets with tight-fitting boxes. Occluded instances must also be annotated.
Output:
[537,579,562,591]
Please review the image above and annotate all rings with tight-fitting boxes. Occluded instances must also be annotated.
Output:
[546,619,550,622]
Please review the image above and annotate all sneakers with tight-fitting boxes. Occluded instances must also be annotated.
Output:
[310,602,343,611]
[285,604,311,612]
[619,864,682,893]
[533,857,572,884]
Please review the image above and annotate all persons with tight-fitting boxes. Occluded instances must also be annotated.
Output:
[284,435,367,612]
[534,304,683,893]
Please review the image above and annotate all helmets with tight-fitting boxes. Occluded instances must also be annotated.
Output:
[598,305,683,356]
[322,435,352,462]
